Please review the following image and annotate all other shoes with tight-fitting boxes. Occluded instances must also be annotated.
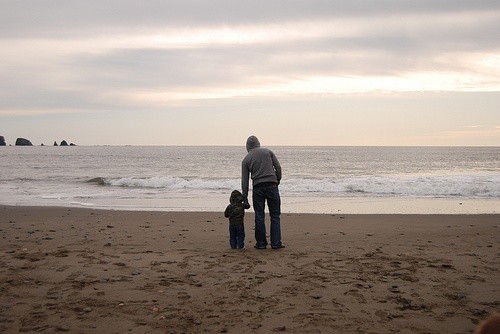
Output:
[272,244,285,249]
[254,245,266,249]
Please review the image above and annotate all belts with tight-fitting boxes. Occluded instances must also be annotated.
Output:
[255,182,278,187]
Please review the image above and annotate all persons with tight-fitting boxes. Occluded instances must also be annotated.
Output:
[242,136,285,249]
[224,190,250,250]
